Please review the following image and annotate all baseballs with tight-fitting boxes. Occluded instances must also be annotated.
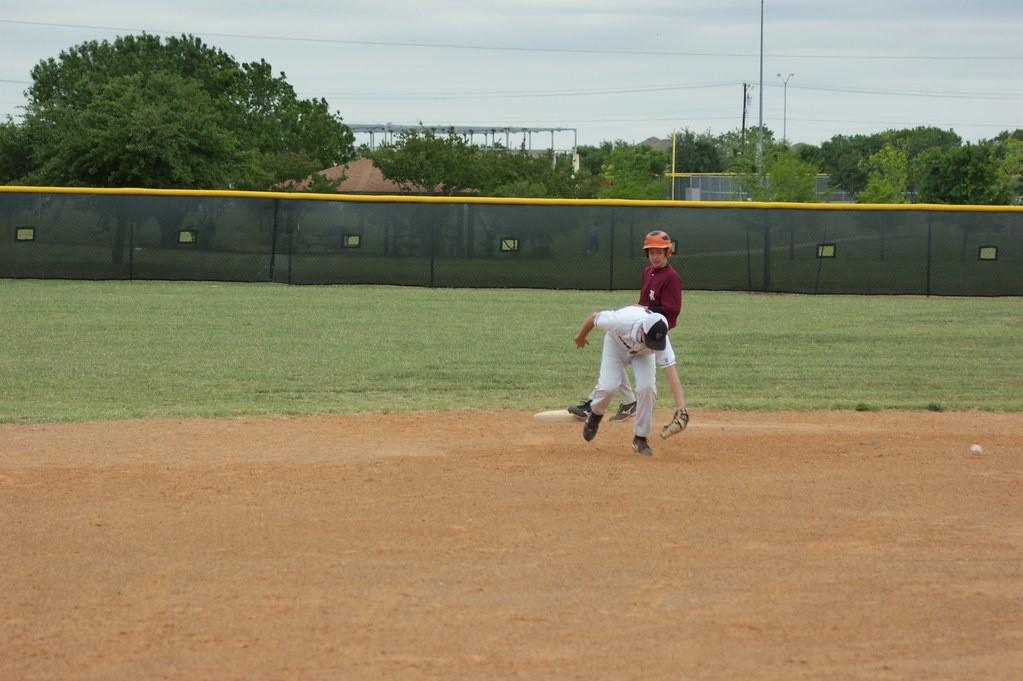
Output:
[970,444,982,455]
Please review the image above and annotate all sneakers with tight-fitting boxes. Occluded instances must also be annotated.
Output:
[567,400,592,420]
[582,412,603,442]
[632,435,653,456]
[609,400,637,422]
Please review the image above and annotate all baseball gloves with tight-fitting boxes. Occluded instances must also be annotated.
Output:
[659,407,689,439]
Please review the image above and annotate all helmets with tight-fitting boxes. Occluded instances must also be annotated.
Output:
[642,231,673,257]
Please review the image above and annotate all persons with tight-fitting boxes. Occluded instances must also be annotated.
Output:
[574,306,689,457]
[589,222,599,254]
[569,230,682,421]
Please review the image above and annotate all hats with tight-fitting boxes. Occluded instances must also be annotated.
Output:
[643,313,669,351]
